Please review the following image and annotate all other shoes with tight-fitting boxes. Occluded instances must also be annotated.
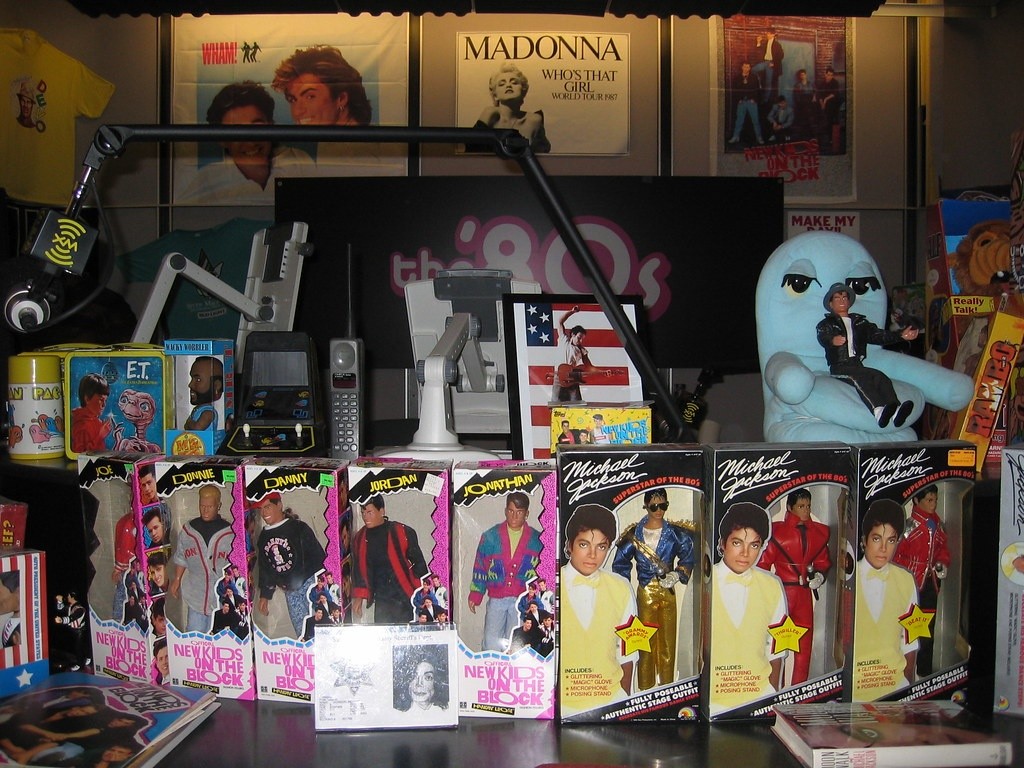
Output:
[769,135,776,141]
[729,137,740,143]
[757,136,764,144]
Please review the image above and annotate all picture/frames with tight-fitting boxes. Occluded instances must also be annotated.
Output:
[500,291,651,458]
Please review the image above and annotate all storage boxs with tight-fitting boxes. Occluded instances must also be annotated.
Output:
[0,199,1024,728]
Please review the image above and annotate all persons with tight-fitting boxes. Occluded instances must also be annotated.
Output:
[55,592,91,671]
[612,487,695,691]
[473,63,552,152]
[337,469,352,609]
[726,27,847,145]
[559,504,640,719]
[469,493,544,653]
[123,463,169,687]
[111,499,138,623]
[816,283,919,427]
[0,687,148,768]
[300,573,344,640]
[212,566,251,639]
[257,492,325,637]
[0,570,22,647]
[171,484,234,634]
[393,651,449,712]
[709,502,789,717]
[550,304,611,404]
[510,579,554,658]
[893,484,951,678]
[852,499,920,703]
[185,356,223,430]
[558,414,612,445]
[412,575,449,629]
[171,42,384,203]
[351,494,429,624]
[757,489,831,686]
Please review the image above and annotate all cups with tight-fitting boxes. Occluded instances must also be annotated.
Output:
[8,356,64,460]
